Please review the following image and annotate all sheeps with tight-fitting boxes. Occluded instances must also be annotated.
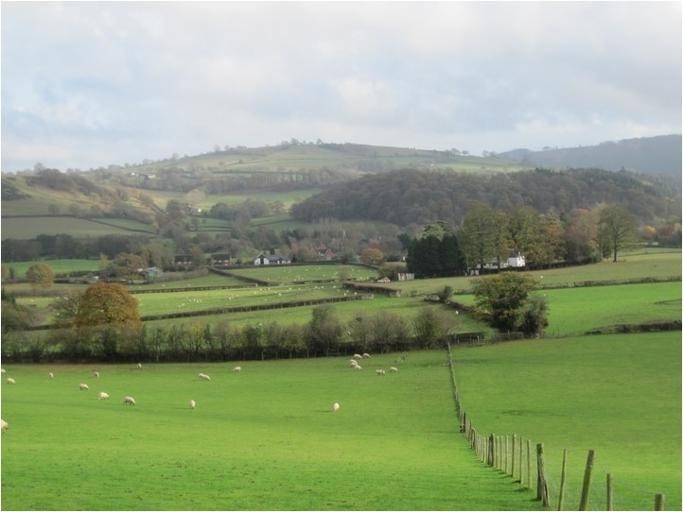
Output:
[1,368,7,375]
[1,417,10,432]
[98,392,111,401]
[49,371,54,379]
[189,399,196,409]
[93,371,99,378]
[352,353,362,360]
[332,401,343,412]
[363,353,371,361]
[124,395,136,407]
[197,372,211,382]
[387,366,399,373]
[231,366,242,375]
[375,367,386,376]
[79,383,89,392]
[349,360,362,371]
[6,377,15,385]
[136,362,142,369]
